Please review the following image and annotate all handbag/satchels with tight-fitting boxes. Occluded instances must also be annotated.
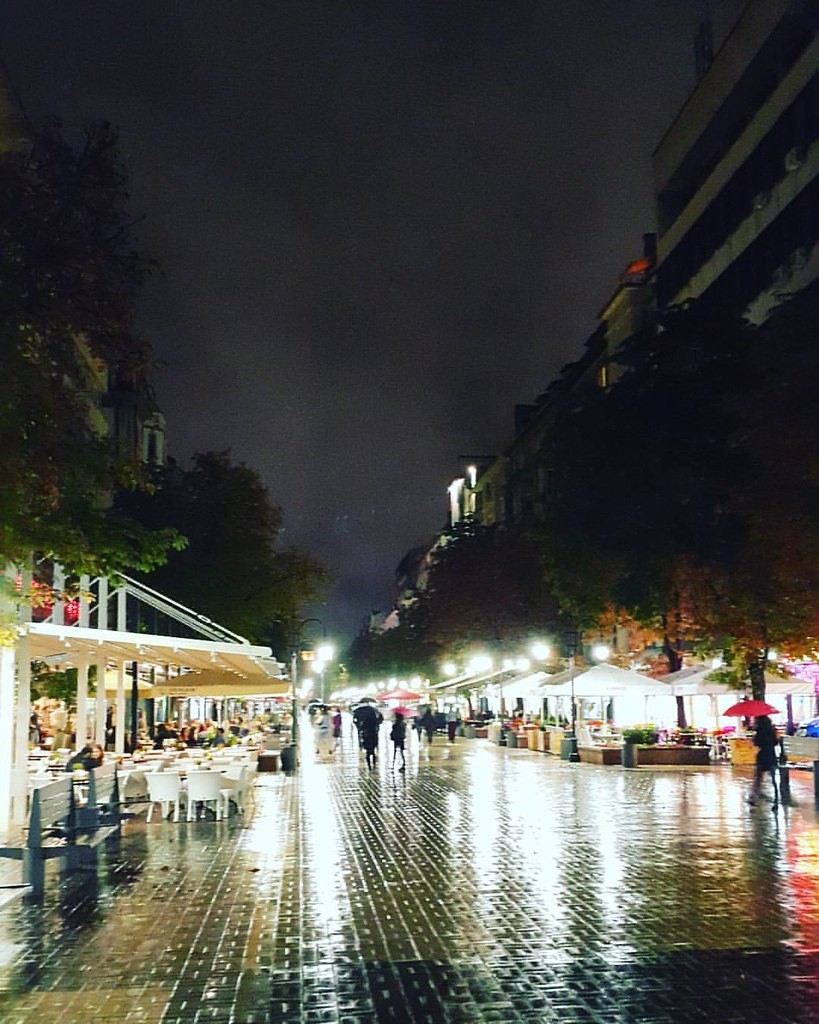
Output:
[779,738,787,766]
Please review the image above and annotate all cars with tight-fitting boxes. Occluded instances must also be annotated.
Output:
[796,719,819,737]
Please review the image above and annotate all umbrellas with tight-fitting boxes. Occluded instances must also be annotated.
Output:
[307,700,330,716]
[150,669,289,725]
[722,700,781,738]
[350,689,425,725]
[85,669,156,705]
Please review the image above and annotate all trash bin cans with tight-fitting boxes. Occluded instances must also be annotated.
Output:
[506,731,517,747]
[560,731,576,760]
[623,744,638,768]
[280,745,295,771]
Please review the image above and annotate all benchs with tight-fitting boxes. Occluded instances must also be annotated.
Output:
[0,761,153,914]
[778,735,819,784]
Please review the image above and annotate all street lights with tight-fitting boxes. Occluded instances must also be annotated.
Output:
[529,615,609,761]
[285,618,331,765]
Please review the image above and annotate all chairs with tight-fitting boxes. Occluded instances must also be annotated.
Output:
[116,748,259,822]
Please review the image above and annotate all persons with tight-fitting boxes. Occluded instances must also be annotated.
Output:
[744,716,779,810]
[314,708,344,754]
[446,704,462,743]
[362,723,378,770]
[30,704,292,753]
[67,744,105,770]
[419,709,436,743]
[391,713,406,772]
[413,717,423,741]
